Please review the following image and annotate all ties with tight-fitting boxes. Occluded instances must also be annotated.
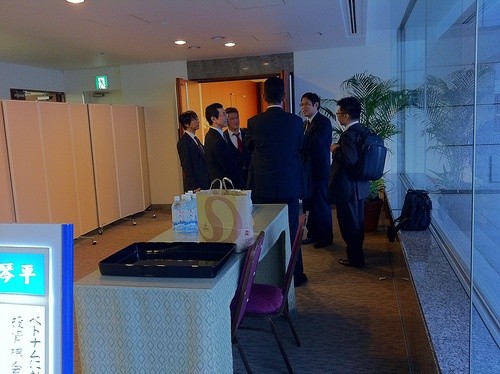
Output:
[194,136,202,152]
[223,133,228,144]
[233,133,242,149]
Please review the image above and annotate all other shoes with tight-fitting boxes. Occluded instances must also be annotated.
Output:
[294,274,308,287]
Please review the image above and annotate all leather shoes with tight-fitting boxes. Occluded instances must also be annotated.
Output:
[338,259,364,268]
[314,240,332,248]
[301,237,314,244]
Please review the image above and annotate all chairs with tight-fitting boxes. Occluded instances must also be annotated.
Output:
[238,211,308,374]
[229,231,264,374]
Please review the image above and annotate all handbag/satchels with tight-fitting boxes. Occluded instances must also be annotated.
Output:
[196,177,255,254]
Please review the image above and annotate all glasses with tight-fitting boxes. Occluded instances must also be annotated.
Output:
[336,112,346,115]
[299,102,314,108]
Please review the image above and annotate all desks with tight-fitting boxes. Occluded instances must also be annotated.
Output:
[73,205,294,374]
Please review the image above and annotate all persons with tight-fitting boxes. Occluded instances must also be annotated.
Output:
[328,97,372,267]
[299,92,334,248]
[204,103,245,189]
[222,107,247,178]
[242,77,307,287]
[177,110,210,193]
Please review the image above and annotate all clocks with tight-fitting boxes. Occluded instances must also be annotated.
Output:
[96,75,108,90]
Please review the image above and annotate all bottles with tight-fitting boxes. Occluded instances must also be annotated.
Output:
[171,190,198,233]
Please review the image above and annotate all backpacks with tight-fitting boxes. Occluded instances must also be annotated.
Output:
[337,128,387,180]
[387,189,432,244]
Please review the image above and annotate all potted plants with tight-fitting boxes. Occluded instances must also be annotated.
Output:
[320,69,417,233]
[418,61,494,209]
